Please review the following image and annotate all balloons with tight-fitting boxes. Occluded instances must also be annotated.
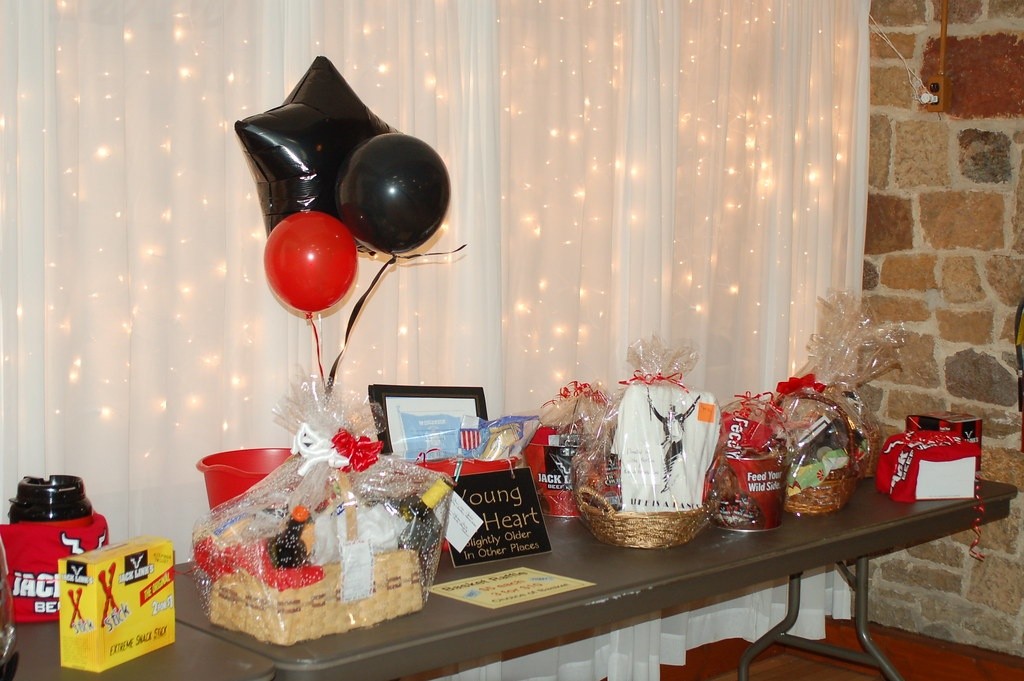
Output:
[265,210,359,319]
[335,133,452,256]
[234,55,404,241]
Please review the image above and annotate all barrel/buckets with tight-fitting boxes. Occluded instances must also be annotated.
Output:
[522,441,595,517]
[195,446,302,513]
[711,456,791,533]
[411,457,519,552]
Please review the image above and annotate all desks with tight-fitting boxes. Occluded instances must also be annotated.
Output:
[0,622,278,681]
[173,471,1021,681]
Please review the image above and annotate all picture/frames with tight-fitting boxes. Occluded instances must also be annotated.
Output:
[368,384,487,463]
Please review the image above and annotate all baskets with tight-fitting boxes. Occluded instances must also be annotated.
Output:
[209,473,421,642]
[766,394,855,516]
[854,429,883,478]
[577,484,718,547]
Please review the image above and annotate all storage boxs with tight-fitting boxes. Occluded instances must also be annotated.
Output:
[58,537,174,672]
[905,411,983,473]
